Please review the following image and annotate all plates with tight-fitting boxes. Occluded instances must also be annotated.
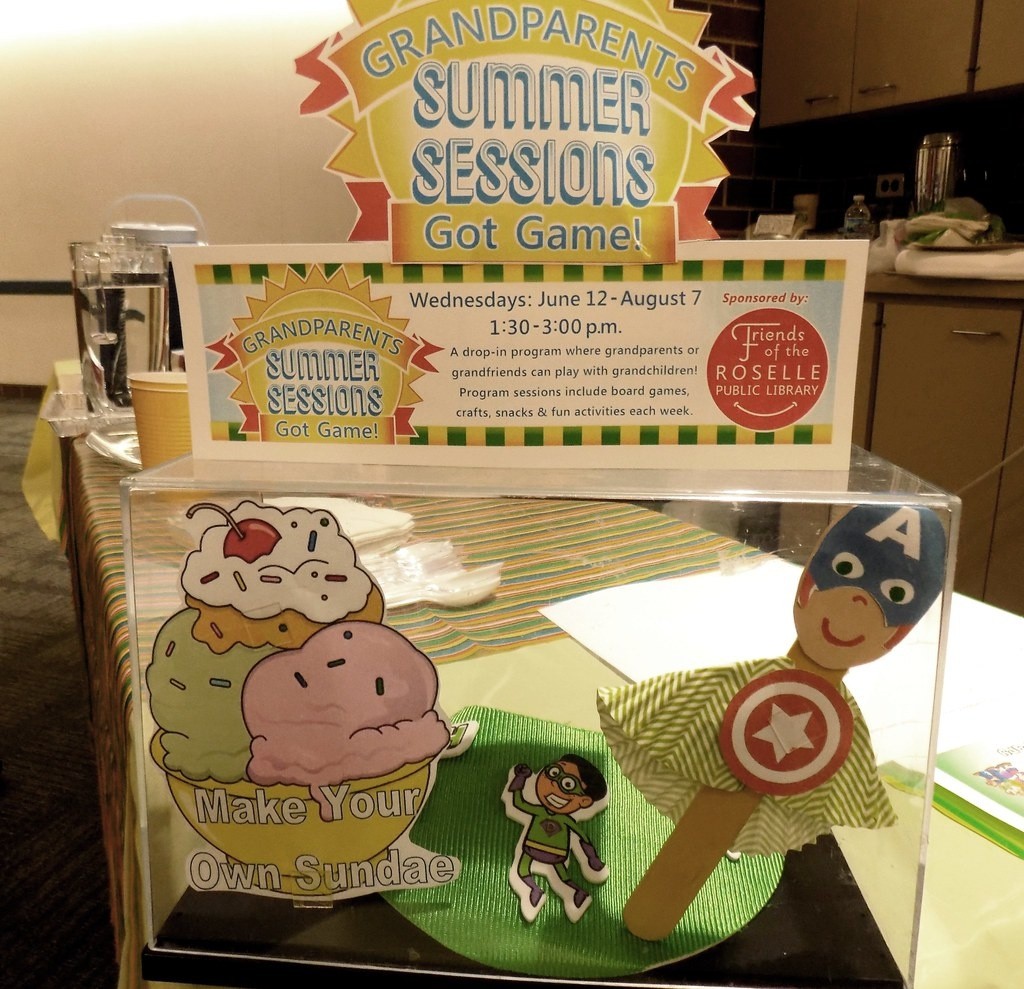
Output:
[86,416,142,471]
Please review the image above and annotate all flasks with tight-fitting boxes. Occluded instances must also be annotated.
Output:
[914,132,957,214]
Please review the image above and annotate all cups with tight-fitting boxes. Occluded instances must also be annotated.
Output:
[70,240,170,414]
[793,194,819,229]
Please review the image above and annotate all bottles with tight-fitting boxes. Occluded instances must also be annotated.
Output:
[843,195,870,230]
[99,194,210,350]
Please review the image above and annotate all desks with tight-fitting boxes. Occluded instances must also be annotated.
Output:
[62,405,1024,989]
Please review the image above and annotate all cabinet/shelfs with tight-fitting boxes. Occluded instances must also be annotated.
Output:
[666,0,1024,238]
[852,301,1024,617]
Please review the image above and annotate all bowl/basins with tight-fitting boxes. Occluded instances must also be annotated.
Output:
[128,372,193,469]
